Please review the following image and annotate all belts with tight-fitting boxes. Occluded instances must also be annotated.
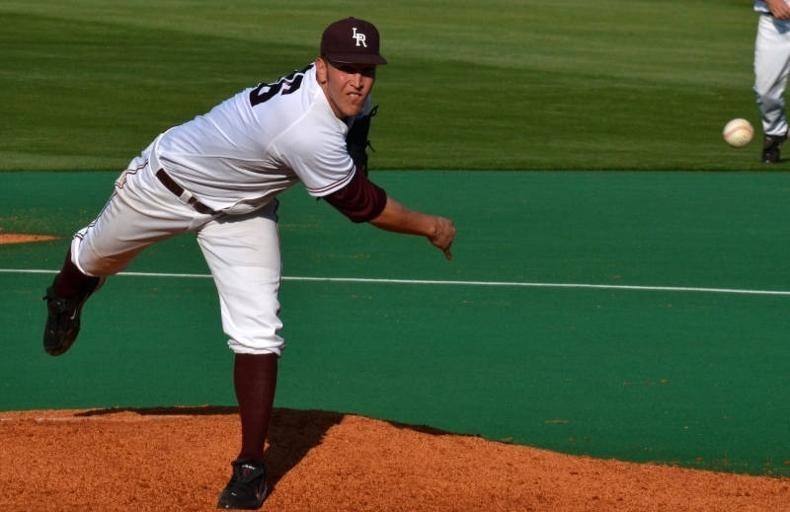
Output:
[155,169,222,216]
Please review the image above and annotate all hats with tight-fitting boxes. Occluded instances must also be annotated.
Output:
[322,16,387,66]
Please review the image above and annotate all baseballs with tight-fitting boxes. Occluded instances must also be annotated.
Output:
[723,118,754,147]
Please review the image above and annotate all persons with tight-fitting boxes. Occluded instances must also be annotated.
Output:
[751,1,790,163]
[41,14,457,512]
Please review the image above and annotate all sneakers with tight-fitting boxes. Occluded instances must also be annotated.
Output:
[41,275,107,357]
[762,134,787,164]
[220,460,268,509]
